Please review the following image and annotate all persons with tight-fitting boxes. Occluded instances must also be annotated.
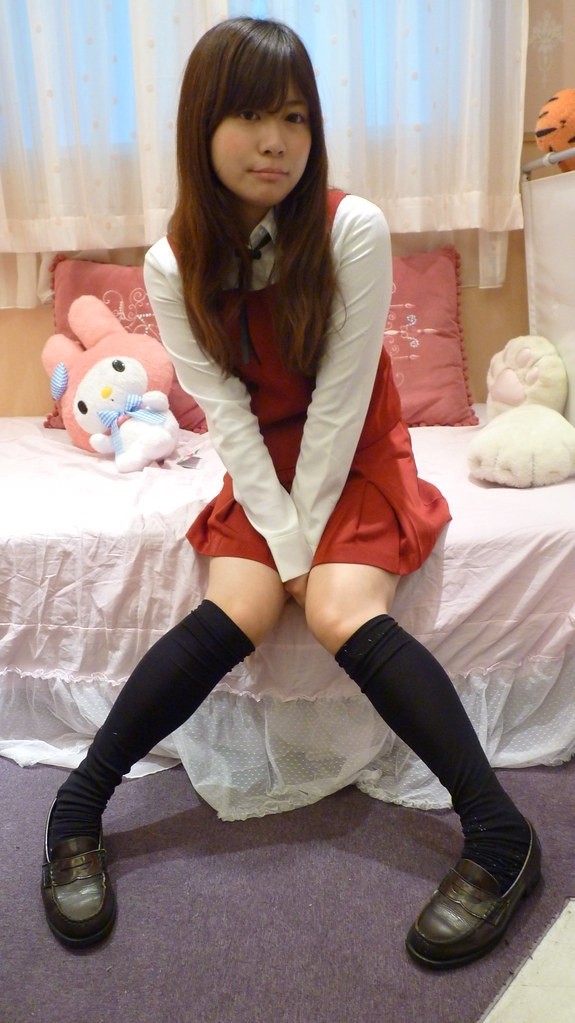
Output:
[41,16,542,971]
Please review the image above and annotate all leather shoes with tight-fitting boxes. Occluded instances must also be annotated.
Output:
[404,819,541,969]
[41,797,118,952]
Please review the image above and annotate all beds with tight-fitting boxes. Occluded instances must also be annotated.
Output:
[0,404,575,823]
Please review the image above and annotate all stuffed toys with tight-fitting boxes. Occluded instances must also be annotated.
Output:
[465,403,575,487]
[484,334,567,420]
[535,88,575,173]
[41,294,178,473]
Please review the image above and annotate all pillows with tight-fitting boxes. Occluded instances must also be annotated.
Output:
[42,245,479,430]
